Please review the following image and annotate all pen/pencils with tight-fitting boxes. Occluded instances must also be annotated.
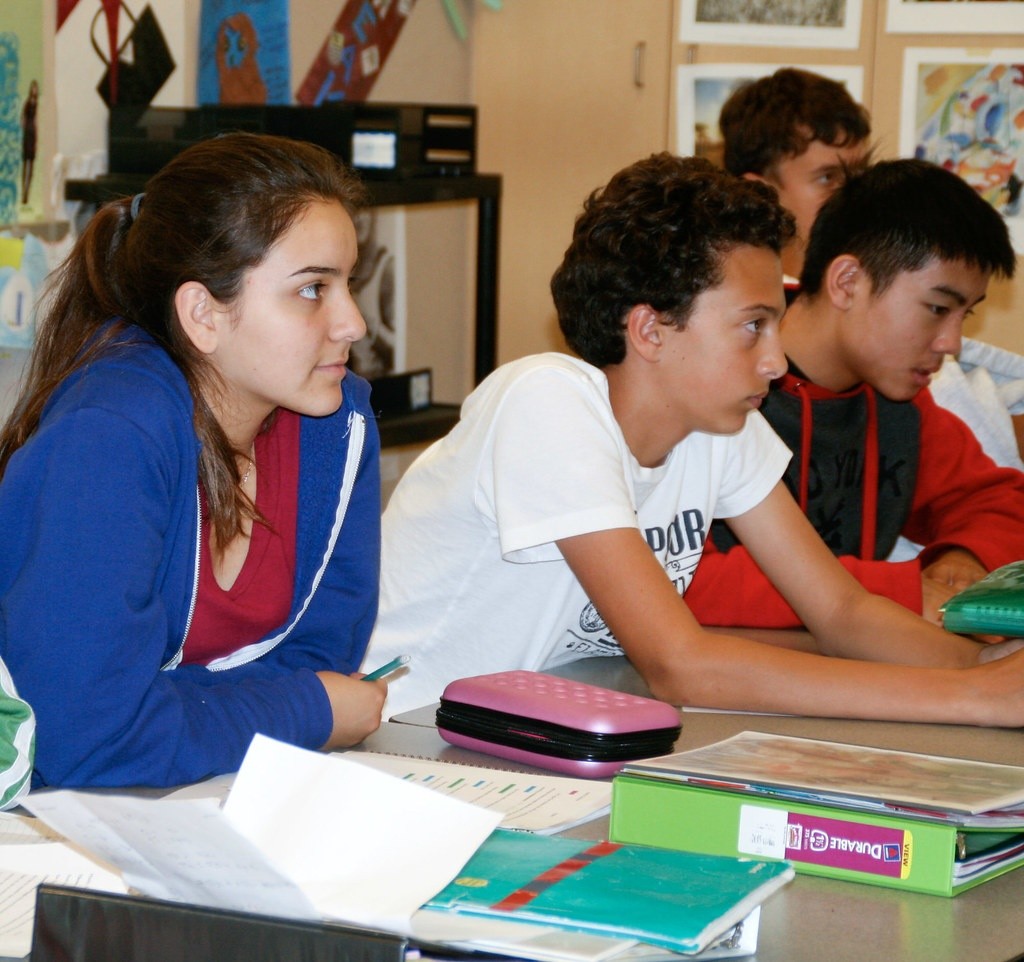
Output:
[361,653,412,681]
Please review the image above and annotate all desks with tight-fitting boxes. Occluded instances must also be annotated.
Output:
[64,171,503,450]
[0,720,1024,962]
[388,623,1024,755]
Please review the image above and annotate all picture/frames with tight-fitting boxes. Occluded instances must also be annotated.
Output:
[678,0,864,52]
[898,47,1024,255]
[885,0,1024,36]
[677,63,866,172]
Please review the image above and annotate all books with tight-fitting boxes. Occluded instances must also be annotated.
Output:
[325,728,1024,962]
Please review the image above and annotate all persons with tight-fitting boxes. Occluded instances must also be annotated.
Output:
[680,159,1023,644]
[718,64,1023,563]
[362,144,1024,733]
[1,129,388,801]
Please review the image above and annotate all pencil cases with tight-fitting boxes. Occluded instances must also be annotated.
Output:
[435,669,684,780]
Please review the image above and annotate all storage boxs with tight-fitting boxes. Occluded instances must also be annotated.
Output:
[106,100,478,180]
